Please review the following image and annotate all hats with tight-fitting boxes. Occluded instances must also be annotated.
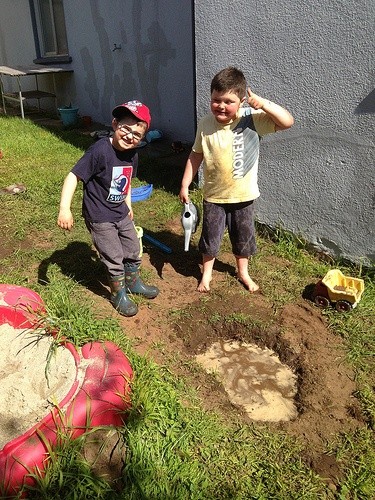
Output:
[112,100,152,132]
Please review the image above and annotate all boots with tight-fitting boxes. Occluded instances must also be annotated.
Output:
[107,273,139,317]
[125,264,159,298]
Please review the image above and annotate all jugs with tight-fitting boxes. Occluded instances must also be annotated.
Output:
[181,202,200,252]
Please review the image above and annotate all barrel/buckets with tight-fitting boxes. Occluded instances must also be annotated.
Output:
[58,107,80,126]
[131,220,144,258]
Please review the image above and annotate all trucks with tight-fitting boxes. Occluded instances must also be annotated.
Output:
[312,268,365,311]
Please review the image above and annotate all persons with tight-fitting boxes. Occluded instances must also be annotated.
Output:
[57,100,159,316]
[179,67,294,293]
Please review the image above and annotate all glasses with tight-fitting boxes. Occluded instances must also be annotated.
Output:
[118,122,142,140]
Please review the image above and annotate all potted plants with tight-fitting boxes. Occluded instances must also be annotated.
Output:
[58,102,80,126]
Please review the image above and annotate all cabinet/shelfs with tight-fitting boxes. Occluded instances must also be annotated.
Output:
[0,65,74,119]
